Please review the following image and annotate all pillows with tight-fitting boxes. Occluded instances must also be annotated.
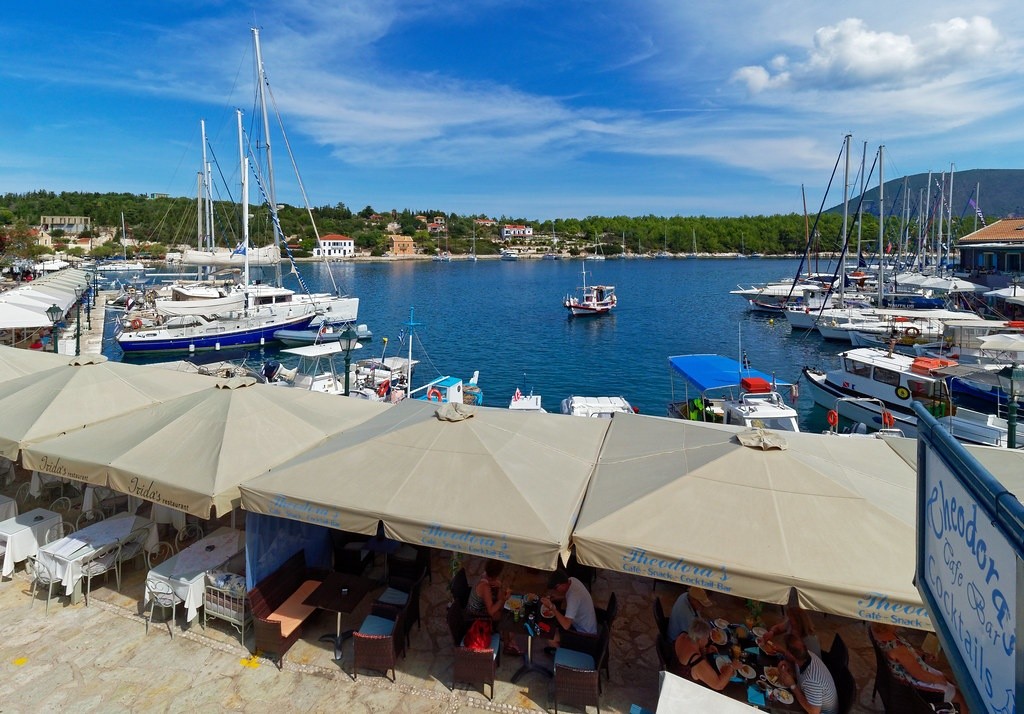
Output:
[207,569,244,599]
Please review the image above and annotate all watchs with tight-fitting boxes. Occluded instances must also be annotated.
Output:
[790,684,797,689]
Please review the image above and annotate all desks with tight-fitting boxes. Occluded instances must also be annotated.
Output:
[38,511,159,605]
[0,495,18,521]
[144,526,246,628]
[709,622,805,713]
[1,508,63,577]
[498,594,555,684]
[302,563,377,651]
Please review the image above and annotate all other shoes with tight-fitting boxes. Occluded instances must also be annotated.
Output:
[502,646,525,656]
[544,646,557,657]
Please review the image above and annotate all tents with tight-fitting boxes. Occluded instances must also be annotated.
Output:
[0,268,96,348]
[0,346,1024,632]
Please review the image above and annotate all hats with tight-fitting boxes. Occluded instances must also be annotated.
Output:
[547,569,568,590]
[688,586,713,607]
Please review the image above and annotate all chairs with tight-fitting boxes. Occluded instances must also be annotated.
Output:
[651,595,669,632]
[327,529,433,683]
[656,632,682,676]
[26,555,62,615]
[49,497,71,517]
[38,473,64,503]
[445,568,503,699]
[556,592,617,714]
[148,542,176,640]
[175,525,204,552]
[821,634,857,714]
[44,522,76,544]
[117,528,150,586]
[14,482,31,512]
[76,509,105,530]
[93,486,117,516]
[867,624,961,714]
[85,543,120,607]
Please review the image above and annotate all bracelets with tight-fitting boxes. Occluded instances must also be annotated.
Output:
[730,664,736,672]
[548,604,556,611]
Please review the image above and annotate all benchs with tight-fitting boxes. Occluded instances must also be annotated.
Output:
[204,566,252,645]
[245,548,329,672]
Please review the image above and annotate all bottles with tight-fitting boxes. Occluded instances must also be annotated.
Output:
[514,608,519,622]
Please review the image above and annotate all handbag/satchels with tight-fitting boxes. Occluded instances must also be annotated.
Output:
[463,619,493,649]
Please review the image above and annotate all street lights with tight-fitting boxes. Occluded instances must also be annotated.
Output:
[95,264,99,295]
[92,268,96,304]
[74,285,83,356]
[84,273,91,323]
[45,304,63,355]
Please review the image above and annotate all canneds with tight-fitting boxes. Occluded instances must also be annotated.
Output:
[520,608,525,617]
[765,685,773,700]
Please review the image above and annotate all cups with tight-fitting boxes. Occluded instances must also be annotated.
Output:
[519,608,525,617]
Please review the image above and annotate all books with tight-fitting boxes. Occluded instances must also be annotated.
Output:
[920,633,941,661]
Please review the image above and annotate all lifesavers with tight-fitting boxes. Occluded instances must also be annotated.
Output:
[883,412,894,426]
[905,327,920,338]
[827,410,838,426]
[850,272,864,276]
[379,380,389,396]
[1009,321,1024,326]
[428,389,442,402]
[132,319,142,329]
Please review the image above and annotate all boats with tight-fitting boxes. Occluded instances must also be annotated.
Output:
[542,254,556,260]
[501,251,518,261]
[562,261,617,316]
[803,344,1024,449]
[273,324,373,346]
[808,307,1024,364]
[278,307,637,415]
[668,321,800,432]
[433,252,451,261]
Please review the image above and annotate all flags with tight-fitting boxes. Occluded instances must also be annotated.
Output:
[512,387,521,402]
[529,387,533,399]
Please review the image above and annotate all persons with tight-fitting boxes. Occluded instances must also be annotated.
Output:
[13,260,37,283]
[460,545,973,714]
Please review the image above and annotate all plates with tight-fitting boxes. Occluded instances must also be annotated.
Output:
[738,664,756,678]
[503,598,524,611]
[755,637,779,655]
[710,627,727,645]
[541,603,556,618]
[728,624,749,635]
[524,595,539,603]
[714,618,729,629]
[756,680,770,691]
[763,667,789,688]
[716,656,737,678]
[753,626,768,637]
[773,688,794,704]
[760,675,767,681]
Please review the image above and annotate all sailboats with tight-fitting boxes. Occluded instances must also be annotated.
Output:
[114,26,359,353]
[586,229,605,260]
[729,134,980,314]
